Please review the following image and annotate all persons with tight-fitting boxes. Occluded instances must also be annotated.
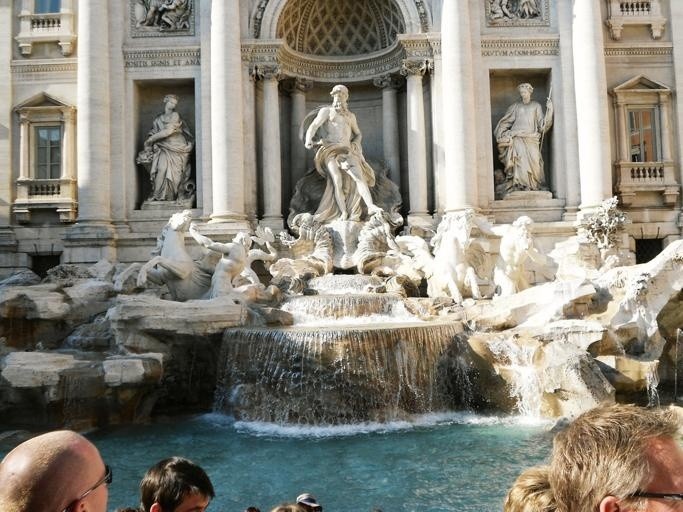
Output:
[146,94,188,201]
[0,429,112,512]
[551,407,681,511]
[501,463,551,511]
[303,83,387,220]
[113,209,545,303]
[138,458,213,510]
[494,81,551,190]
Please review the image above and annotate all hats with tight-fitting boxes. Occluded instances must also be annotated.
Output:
[296,493,322,512]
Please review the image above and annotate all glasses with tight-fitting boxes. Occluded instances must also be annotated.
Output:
[65,464,112,512]
